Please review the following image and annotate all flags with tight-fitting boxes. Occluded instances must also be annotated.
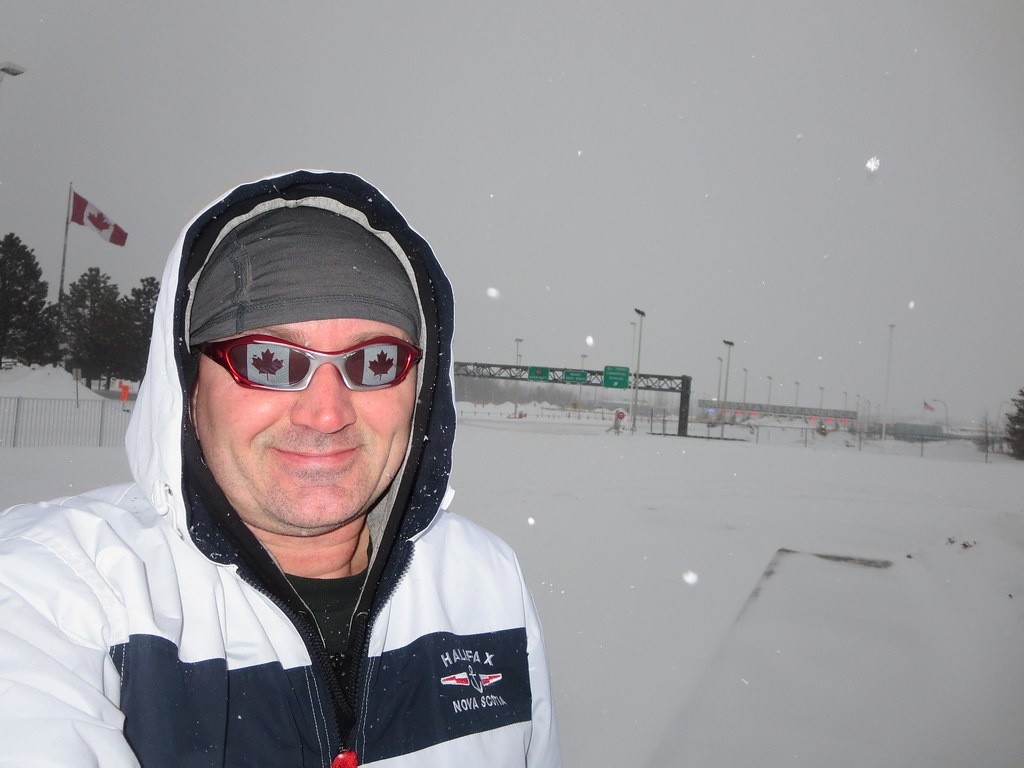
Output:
[924,402,935,412]
[70,192,128,247]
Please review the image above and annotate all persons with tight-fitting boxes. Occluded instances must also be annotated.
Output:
[0,168,561,768]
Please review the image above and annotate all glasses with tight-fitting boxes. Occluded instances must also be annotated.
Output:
[193,333,424,392]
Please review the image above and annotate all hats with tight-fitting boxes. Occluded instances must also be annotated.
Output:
[188,205,422,346]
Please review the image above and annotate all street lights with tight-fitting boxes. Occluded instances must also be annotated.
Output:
[715,338,733,439]
[630,307,647,434]
[793,381,804,442]
[576,353,589,419]
[818,387,824,426]
[513,337,524,418]
[765,375,774,442]
[741,367,749,410]
